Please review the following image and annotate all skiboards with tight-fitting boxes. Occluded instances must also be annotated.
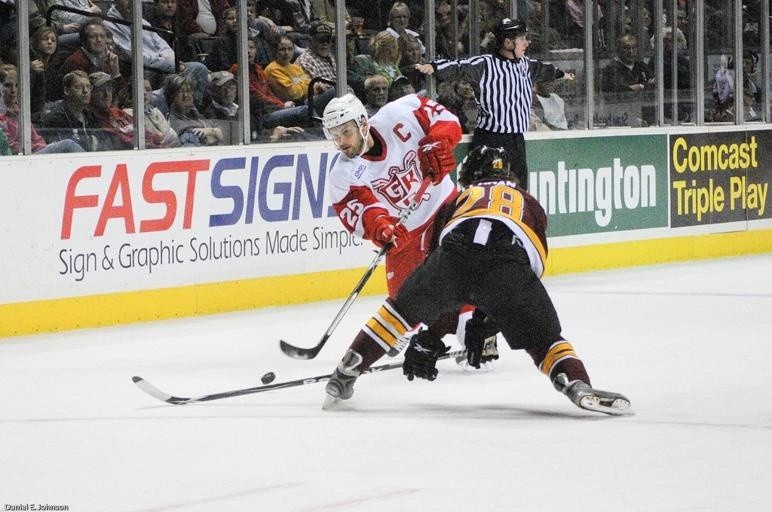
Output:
[280,175,432,360]
[132,351,466,406]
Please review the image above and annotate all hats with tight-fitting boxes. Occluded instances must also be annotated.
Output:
[309,24,332,36]
[212,71,238,87]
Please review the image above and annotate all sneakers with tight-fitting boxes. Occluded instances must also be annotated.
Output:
[565,379,630,416]
[326,366,355,401]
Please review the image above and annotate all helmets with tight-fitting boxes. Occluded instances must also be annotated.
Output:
[495,18,527,37]
[460,143,512,183]
[323,94,368,140]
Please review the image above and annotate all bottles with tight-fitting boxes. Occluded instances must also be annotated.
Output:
[0,128,12,156]
[71,129,81,144]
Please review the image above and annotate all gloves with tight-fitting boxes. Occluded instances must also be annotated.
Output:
[362,205,411,255]
[463,319,500,368]
[417,141,457,185]
[403,331,446,382]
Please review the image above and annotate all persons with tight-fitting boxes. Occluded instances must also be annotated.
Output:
[323,94,502,371]
[320,144,631,417]
